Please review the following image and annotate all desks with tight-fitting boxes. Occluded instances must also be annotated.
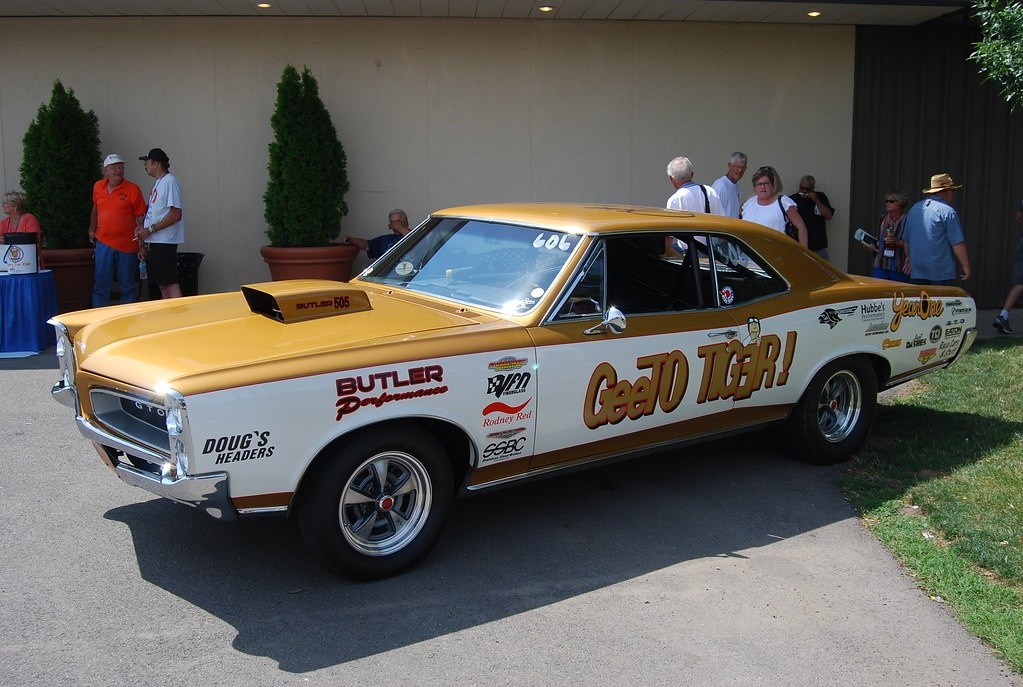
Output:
[0,270,59,358]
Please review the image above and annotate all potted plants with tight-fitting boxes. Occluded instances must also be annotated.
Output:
[18,76,106,315]
[258,62,360,283]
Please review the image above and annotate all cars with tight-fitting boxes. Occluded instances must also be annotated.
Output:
[46,203,978,582]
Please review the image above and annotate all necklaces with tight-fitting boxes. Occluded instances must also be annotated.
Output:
[890,211,903,226]
[7,213,23,232]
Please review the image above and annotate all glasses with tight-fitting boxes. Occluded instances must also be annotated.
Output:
[756,183,774,189]
[885,199,897,203]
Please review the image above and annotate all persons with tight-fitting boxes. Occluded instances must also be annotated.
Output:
[902,173,971,287]
[741,166,808,279]
[0,191,45,270]
[710,152,748,257]
[785,175,835,262]
[993,195,1023,335]
[89,153,146,308]
[343,209,427,278]
[864,190,907,282]
[664,157,725,257]
[134,148,184,299]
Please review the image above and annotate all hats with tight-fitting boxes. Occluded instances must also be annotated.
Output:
[921,173,963,193]
[139,148,169,164]
[104,154,125,167]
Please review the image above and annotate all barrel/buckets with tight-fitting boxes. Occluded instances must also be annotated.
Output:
[3,231,38,274]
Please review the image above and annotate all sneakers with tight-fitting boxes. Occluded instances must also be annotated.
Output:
[993,316,1014,335]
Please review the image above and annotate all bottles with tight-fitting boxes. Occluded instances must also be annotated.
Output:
[139,258,147,279]
[38,256,41,273]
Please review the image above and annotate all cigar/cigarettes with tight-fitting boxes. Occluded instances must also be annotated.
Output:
[132,237,138,241]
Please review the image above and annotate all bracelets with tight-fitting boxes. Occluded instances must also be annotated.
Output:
[900,240,904,247]
[147,224,156,233]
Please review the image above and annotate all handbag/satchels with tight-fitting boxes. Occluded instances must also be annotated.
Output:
[777,194,798,241]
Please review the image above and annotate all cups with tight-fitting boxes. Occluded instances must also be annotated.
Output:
[886,228,893,239]
